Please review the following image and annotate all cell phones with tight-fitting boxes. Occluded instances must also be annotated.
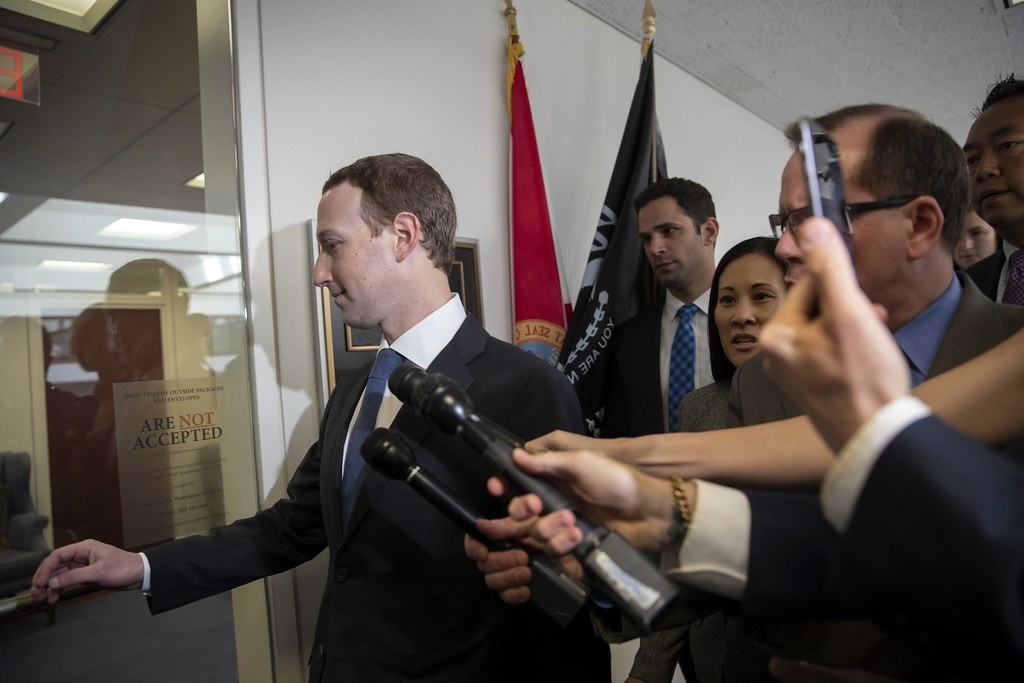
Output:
[795,117,853,262]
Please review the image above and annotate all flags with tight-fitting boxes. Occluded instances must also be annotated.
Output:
[505,33,573,368]
[554,38,669,439]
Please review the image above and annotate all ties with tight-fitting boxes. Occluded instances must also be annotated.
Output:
[667,303,700,436]
[1001,249,1024,307]
[343,347,407,530]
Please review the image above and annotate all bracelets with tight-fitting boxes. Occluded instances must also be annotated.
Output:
[656,473,691,523]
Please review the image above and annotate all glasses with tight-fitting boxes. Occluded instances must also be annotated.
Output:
[768,194,947,241]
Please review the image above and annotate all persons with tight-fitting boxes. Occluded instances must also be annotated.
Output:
[960,71,1024,308]
[486,224,1024,683]
[954,211,1003,272]
[572,177,719,439]
[686,103,1024,683]
[623,235,794,683]
[0,219,382,683]
[30,152,612,683]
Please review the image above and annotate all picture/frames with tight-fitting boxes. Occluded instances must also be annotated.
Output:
[318,238,482,399]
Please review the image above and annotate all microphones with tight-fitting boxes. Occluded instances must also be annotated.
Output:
[359,363,683,631]
